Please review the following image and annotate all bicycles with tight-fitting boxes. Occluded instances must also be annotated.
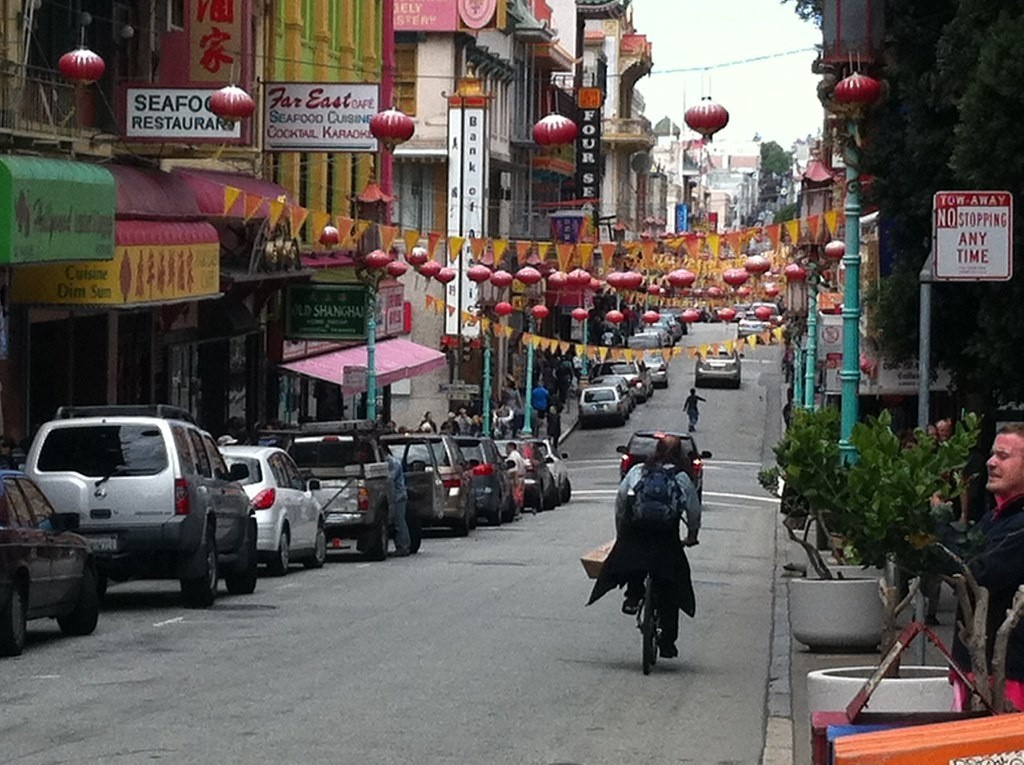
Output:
[629,538,701,676]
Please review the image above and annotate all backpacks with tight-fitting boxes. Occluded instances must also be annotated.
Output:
[621,458,695,538]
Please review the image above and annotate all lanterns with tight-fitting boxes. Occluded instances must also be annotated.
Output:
[60,47,104,88]
[209,86,254,121]
[684,96,729,140]
[319,227,845,328]
[534,112,577,149]
[834,72,878,109]
[370,110,414,150]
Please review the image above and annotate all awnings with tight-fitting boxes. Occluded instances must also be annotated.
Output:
[0,153,221,309]
[276,337,447,393]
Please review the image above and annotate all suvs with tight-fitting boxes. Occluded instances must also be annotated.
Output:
[25,404,259,610]
[448,433,516,527]
[285,418,447,562]
[380,431,480,539]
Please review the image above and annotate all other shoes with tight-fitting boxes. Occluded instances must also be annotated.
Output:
[690,425,695,432]
[622,583,646,615]
[659,644,678,658]
[689,428,693,432]
[394,548,411,557]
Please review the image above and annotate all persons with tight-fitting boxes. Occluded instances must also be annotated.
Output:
[504,442,526,519]
[587,436,700,659]
[377,445,410,557]
[895,418,1024,715]
[683,388,705,432]
[376,406,483,440]
[491,307,638,450]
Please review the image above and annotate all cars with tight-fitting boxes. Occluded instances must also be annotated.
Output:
[0,468,102,657]
[493,439,559,512]
[215,443,328,575]
[692,348,745,388]
[617,430,713,508]
[578,307,689,429]
[531,440,572,507]
[714,301,780,345]
